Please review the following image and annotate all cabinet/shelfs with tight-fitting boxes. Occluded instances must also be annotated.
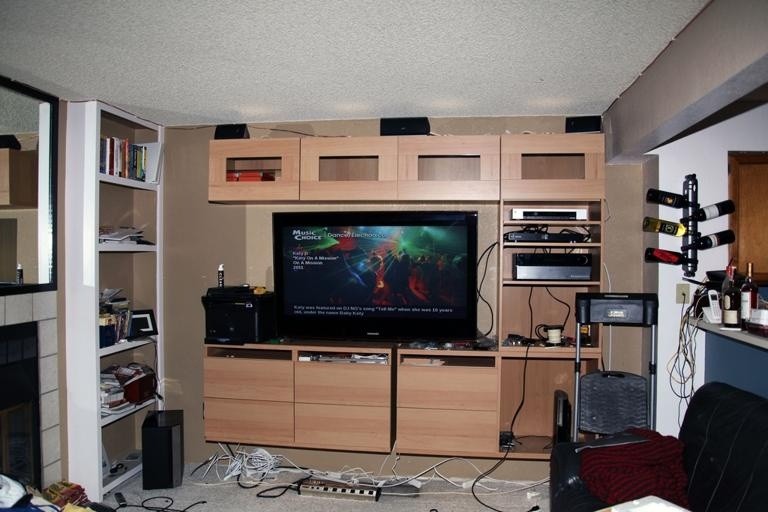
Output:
[501,133,605,199]
[66,97,165,504]
[395,346,499,457]
[398,135,501,202]
[499,199,601,459]
[202,343,294,448]
[207,137,300,205]
[294,341,396,454]
[299,136,398,203]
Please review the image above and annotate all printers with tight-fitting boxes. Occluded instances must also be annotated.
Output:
[200,283,274,346]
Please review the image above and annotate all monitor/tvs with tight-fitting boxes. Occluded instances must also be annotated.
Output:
[270,211,480,347]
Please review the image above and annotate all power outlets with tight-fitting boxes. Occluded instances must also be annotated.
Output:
[676,283,690,304]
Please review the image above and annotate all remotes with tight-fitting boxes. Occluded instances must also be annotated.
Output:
[115,491,125,510]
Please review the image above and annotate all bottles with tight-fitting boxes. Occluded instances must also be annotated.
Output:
[641,172,760,328]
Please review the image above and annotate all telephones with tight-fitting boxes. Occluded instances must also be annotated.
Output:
[702,289,722,324]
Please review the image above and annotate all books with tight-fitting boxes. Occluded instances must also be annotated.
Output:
[99,359,155,419]
[98,134,164,185]
[98,224,145,245]
[98,288,134,346]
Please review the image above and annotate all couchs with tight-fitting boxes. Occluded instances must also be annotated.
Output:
[549,380,768,512]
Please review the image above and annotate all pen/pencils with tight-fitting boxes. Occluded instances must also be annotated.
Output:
[719,327,742,331]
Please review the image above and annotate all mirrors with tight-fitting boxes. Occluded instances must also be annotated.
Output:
[0,74,59,297]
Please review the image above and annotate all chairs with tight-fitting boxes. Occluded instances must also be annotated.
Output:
[572,291,659,443]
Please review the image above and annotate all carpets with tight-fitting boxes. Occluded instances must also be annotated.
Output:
[102,463,550,512]
